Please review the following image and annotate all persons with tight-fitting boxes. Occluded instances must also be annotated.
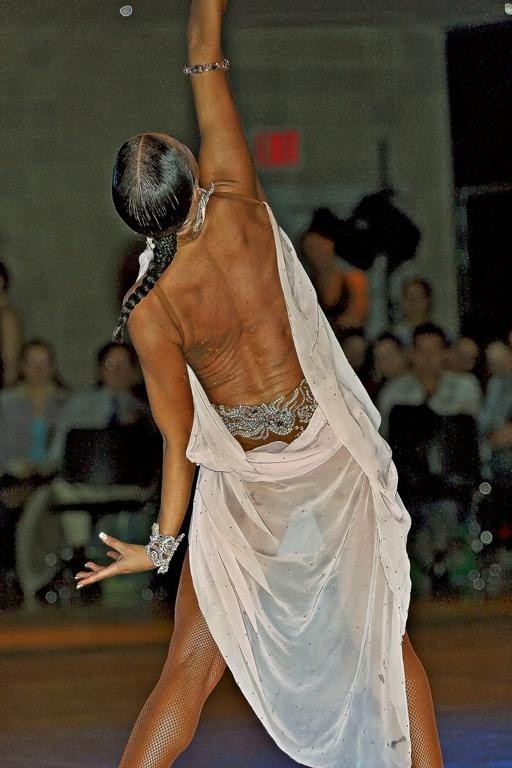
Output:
[76,0,458,768]
[0,261,163,604]
[297,226,512,593]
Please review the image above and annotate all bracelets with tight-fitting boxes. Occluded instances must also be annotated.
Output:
[147,522,184,575]
[179,56,229,74]
